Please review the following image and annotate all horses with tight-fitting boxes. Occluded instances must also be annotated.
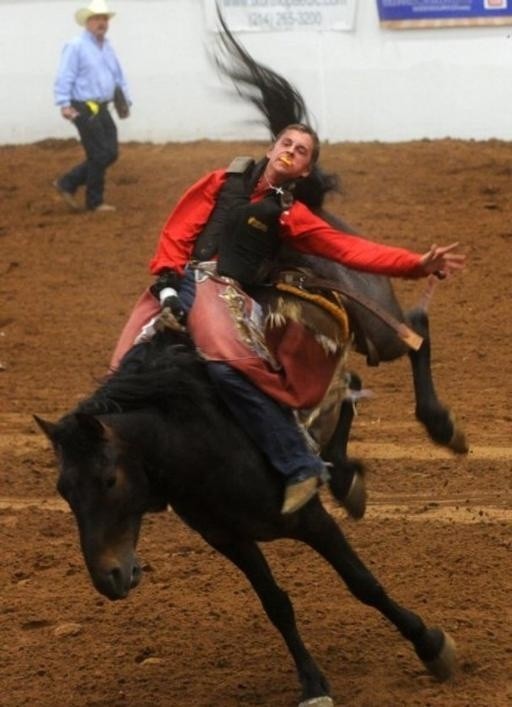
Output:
[30,0,474,707]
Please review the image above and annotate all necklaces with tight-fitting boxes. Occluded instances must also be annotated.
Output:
[263,169,291,196]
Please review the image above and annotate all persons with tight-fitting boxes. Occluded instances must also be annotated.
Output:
[51,2,130,212]
[109,123,468,516]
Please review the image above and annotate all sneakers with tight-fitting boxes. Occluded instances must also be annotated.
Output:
[278,473,327,514]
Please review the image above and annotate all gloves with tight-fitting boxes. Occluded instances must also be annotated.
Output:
[159,287,184,316]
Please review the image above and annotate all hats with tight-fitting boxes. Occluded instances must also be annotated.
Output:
[74,0,113,21]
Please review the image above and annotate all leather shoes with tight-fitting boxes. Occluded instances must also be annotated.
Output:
[97,202,116,212]
[53,176,80,213]
